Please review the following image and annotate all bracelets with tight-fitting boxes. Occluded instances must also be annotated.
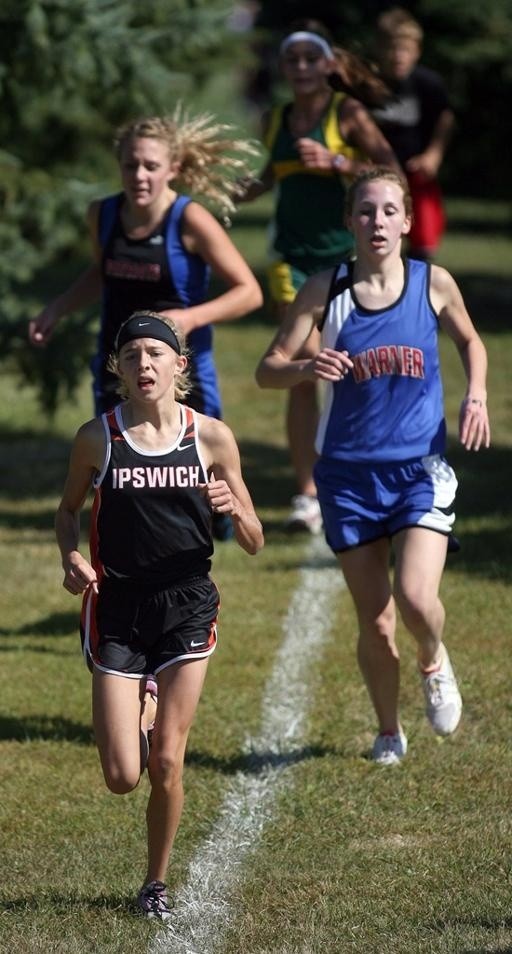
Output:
[331,153,345,172]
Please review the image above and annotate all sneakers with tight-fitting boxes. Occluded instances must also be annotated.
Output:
[145,672,158,767]
[285,494,324,537]
[372,726,409,767]
[418,636,463,738]
[140,877,172,923]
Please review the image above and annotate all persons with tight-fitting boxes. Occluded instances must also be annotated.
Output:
[346,7,456,264]
[54,309,266,923]
[233,21,411,534]
[253,168,490,767]
[28,99,267,544]
